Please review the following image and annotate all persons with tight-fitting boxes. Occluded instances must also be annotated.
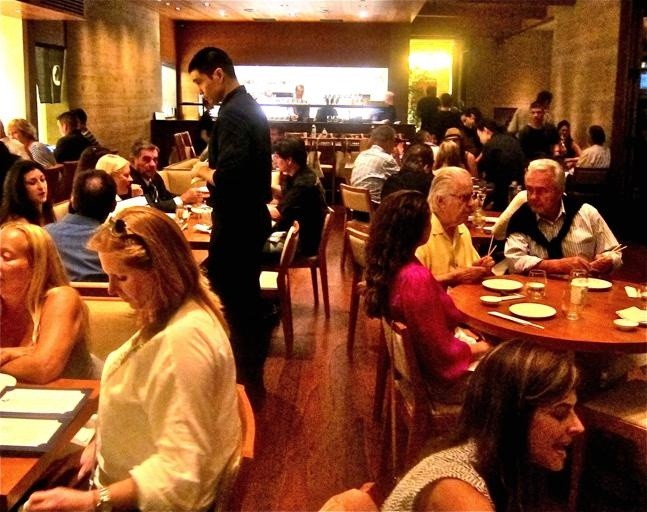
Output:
[290,85,309,116]
[410,131,440,168]
[0,160,57,228]
[195,97,215,156]
[128,139,203,212]
[557,120,580,158]
[416,85,440,131]
[575,125,608,168]
[460,107,486,133]
[7,118,57,170]
[478,116,524,209]
[432,140,472,167]
[381,338,585,512]
[21,206,241,512]
[508,91,557,135]
[1,120,32,163]
[414,166,494,285]
[443,127,478,174]
[266,136,325,257]
[44,170,117,281]
[350,124,401,205]
[438,93,458,128]
[271,139,290,196]
[380,142,434,197]
[363,190,494,383]
[505,159,623,275]
[53,111,92,163]
[374,92,396,122]
[189,47,273,399]
[95,154,133,202]
[71,108,102,146]
[0,223,97,384]
[519,102,553,160]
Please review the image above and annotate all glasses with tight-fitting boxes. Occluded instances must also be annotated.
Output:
[450,192,479,200]
[106,213,129,234]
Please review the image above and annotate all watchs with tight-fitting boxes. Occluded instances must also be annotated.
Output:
[96,487,113,511]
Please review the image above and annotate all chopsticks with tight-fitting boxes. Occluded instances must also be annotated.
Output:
[488,311,545,329]
[480,234,497,266]
[588,243,627,266]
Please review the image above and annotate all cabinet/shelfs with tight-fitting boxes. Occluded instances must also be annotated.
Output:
[256,94,398,131]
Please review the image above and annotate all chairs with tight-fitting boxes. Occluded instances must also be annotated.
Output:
[379,320,469,477]
[568,378,647,508]
[65,160,78,181]
[74,292,146,383]
[339,184,374,272]
[50,164,63,186]
[219,387,260,512]
[174,129,194,156]
[291,205,334,317]
[329,151,345,205]
[345,219,374,334]
[260,220,301,357]
[317,138,345,205]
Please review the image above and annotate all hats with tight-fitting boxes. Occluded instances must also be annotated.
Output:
[444,128,461,137]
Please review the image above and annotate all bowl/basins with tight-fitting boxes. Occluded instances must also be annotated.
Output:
[480,295,502,305]
[613,319,639,331]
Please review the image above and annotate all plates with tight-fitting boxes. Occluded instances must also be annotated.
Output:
[620,310,647,325]
[508,302,557,317]
[571,278,612,290]
[482,278,523,290]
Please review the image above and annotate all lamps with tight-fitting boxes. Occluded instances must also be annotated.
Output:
[220,11,224,16]
[256,0,325,21]
[360,11,369,19]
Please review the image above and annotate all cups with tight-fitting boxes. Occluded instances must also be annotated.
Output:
[637,283,647,309]
[569,269,588,293]
[525,269,547,300]
[561,285,587,320]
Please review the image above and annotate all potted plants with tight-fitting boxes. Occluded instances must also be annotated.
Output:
[408,67,438,130]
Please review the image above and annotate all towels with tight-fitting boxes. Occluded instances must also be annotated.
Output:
[614,305,647,325]
